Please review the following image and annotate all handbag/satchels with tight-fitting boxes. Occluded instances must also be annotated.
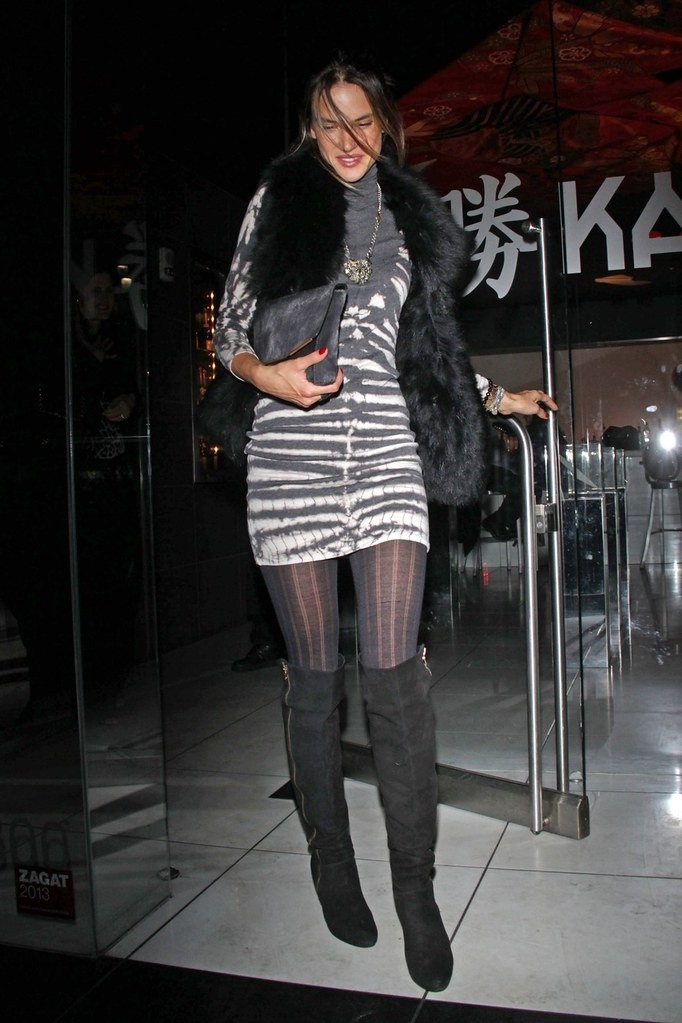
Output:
[251,282,347,385]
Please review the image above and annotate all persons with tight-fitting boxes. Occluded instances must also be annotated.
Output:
[213,53,558,991]
[196,286,292,671]
[481,414,568,542]
[31,262,143,711]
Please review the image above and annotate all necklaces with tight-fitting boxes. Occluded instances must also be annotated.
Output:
[342,182,382,284]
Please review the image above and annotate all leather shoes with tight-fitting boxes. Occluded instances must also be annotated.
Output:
[232,641,282,672]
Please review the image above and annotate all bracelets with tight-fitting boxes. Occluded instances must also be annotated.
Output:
[483,378,505,416]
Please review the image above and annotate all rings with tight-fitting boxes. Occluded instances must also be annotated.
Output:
[319,394,331,401]
[515,441,518,442]
[505,440,509,442]
[120,413,124,419]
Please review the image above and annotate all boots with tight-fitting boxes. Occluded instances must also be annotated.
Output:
[356,643,455,993]
[278,653,378,948]
